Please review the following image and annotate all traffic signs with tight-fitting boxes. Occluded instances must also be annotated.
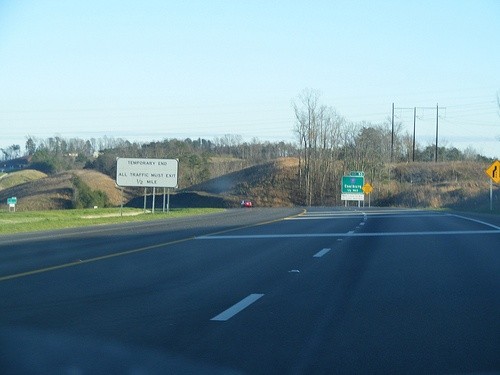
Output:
[115,157,179,188]
[340,175,365,194]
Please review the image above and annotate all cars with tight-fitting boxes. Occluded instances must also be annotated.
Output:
[240,200,252,208]
